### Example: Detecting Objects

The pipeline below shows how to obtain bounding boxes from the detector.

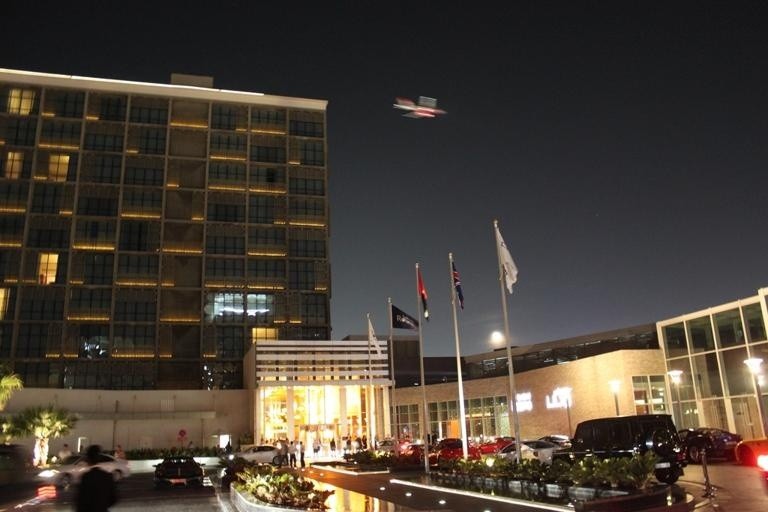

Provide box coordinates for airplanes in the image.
[393,95,448,120]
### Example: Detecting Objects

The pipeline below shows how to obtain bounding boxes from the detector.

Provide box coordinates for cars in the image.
[223,445,288,466]
[402,435,572,466]
[37,452,132,490]
[677,427,742,465]
[153,456,206,489]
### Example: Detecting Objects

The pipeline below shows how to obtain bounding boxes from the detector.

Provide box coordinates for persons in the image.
[299,440,305,468]
[114,443,126,459]
[59,443,72,461]
[65,444,118,512]
[288,440,298,467]
[275,441,289,468]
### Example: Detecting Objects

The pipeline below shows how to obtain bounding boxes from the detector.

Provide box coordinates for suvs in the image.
[574,414,688,485]
[367,440,401,454]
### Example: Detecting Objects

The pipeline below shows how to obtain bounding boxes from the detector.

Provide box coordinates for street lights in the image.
[742,357,768,437]
[558,386,572,435]
[665,368,685,429]
[608,380,620,415]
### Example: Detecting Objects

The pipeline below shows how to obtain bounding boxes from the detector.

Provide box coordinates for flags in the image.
[496,227,519,293]
[391,304,418,331]
[418,269,430,321]
[369,319,380,356]
[452,257,464,310]
